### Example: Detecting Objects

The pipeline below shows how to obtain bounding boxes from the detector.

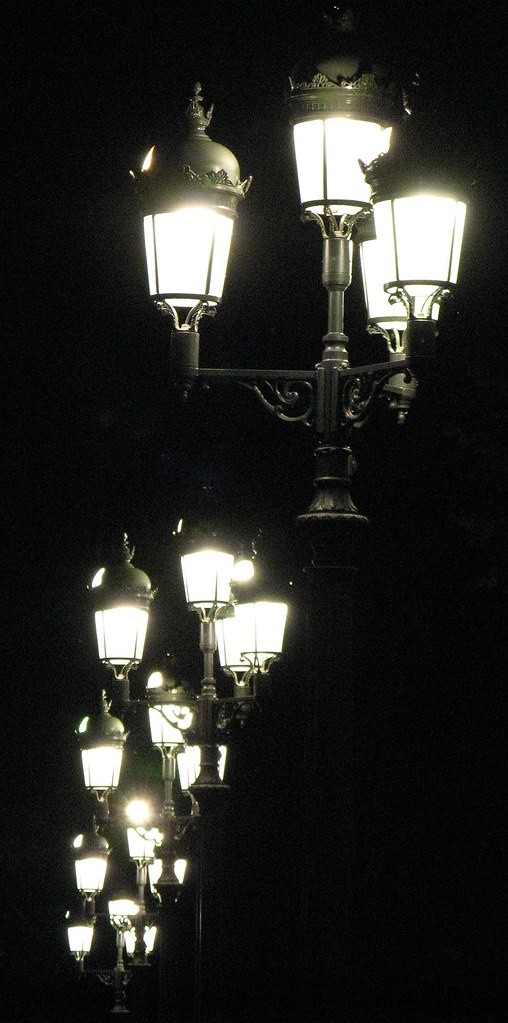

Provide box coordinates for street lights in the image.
[67,9,478,1023]
